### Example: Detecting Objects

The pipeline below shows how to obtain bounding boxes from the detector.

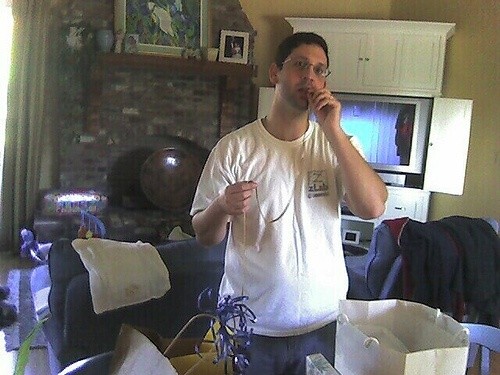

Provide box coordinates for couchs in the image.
[345,217,500,300]
[30,241,228,375]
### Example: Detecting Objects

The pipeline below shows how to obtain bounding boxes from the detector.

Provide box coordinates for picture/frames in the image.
[218,30,250,64]
[113,0,208,59]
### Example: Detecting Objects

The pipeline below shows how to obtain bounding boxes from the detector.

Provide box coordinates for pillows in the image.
[49,239,87,322]
[364,223,402,298]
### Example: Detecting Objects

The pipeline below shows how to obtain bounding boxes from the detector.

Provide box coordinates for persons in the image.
[189,32,388,375]
[227,38,242,60]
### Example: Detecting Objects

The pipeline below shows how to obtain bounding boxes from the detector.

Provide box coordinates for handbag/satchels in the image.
[337,299,469,375]
[109,313,234,375]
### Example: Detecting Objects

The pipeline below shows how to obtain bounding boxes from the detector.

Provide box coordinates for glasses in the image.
[283,58,331,80]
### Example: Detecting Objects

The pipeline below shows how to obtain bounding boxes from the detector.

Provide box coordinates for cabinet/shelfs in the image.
[258,17,474,258]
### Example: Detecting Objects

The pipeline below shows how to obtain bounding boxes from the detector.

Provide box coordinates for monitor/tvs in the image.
[309,92,433,188]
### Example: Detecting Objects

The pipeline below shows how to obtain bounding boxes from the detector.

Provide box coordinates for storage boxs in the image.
[306,353,341,375]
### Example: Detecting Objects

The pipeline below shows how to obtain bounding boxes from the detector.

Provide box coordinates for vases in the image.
[96,21,114,51]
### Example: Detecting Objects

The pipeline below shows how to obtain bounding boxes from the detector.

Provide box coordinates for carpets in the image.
[3,269,48,352]
